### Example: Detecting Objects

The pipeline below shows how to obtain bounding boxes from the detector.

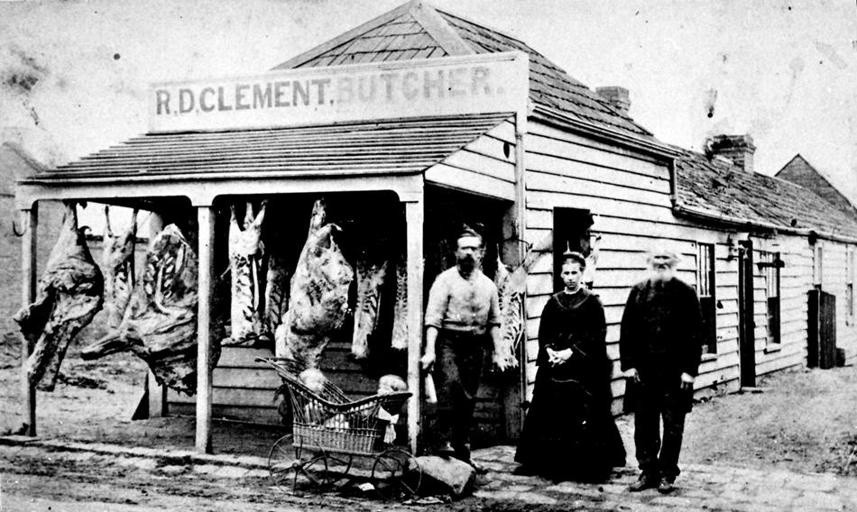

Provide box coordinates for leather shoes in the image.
[631,471,658,492]
[468,459,489,474]
[438,442,455,456]
[659,476,673,492]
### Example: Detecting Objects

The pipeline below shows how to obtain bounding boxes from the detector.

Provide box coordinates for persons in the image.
[619,243,705,494]
[513,252,626,485]
[417,231,514,462]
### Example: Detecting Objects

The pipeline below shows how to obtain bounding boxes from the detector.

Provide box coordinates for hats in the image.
[562,251,586,268]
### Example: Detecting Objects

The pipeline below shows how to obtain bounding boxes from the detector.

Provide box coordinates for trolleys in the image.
[255,356,422,501]
